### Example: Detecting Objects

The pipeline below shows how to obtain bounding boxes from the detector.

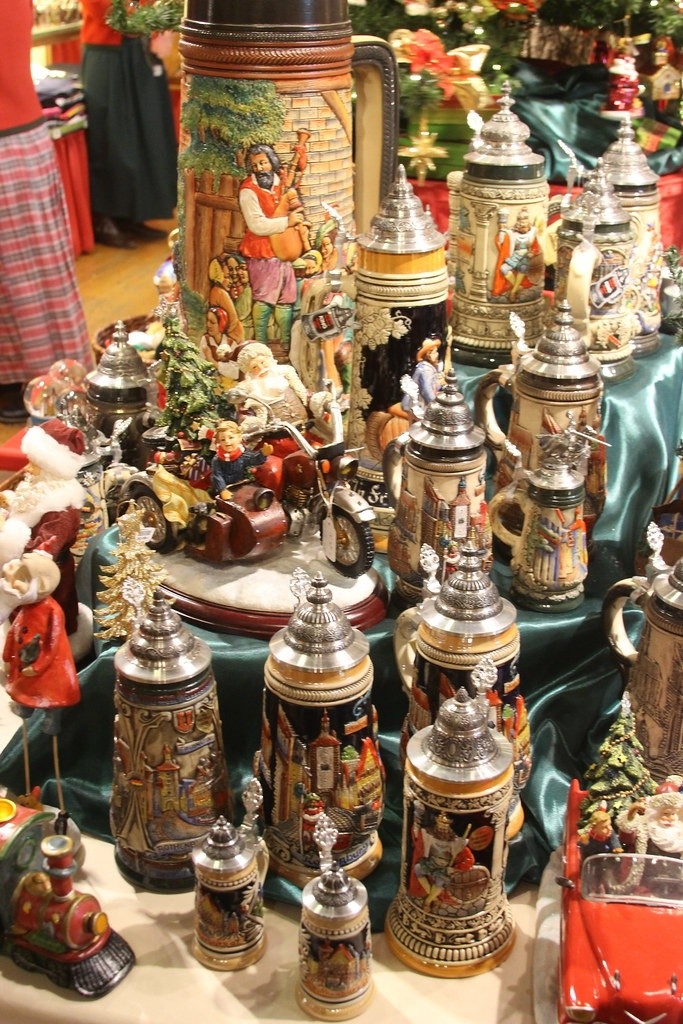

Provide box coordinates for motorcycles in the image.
[117,378,378,580]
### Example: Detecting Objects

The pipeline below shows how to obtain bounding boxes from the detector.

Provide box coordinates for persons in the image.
[1,552,80,736]
[76,1,179,249]
[401,338,445,412]
[606,49,642,112]
[221,338,336,450]
[1,1,98,426]
[617,773,683,901]
[1,416,89,642]
[210,420,274,499]
[575,810,624,895]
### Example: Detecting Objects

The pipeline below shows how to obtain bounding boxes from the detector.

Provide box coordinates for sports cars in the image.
[555,780,683,1024]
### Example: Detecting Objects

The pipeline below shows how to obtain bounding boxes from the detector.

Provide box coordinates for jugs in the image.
[87,0,683,977]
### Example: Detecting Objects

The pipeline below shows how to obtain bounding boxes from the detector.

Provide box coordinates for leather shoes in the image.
[94,215,137,247]
[0,391,30,424]
[113,215,167,240]
